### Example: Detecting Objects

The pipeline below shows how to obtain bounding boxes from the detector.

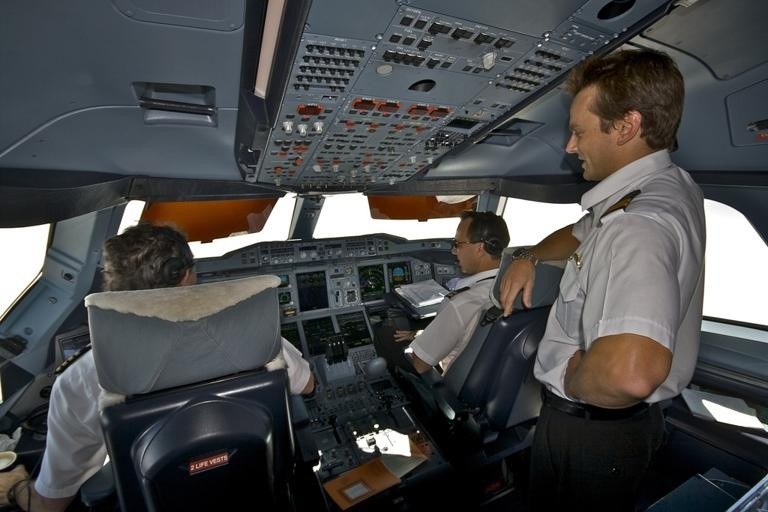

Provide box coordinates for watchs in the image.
[512,248,538,266]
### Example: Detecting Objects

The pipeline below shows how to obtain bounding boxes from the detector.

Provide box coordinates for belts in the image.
[544,389,650,420]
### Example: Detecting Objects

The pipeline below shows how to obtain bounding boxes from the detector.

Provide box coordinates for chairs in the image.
[642,418,768,511]
[77,276,305,512]
[399,247,570,506]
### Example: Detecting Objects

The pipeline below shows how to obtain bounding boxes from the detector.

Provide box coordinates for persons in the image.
[499,48,706,512]
[0,223,314,512]
[393,211,510,374]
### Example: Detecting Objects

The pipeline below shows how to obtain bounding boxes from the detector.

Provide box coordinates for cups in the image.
[0,452,17,472]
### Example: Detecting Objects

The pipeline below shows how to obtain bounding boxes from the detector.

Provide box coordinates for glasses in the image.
[451,239,485,248]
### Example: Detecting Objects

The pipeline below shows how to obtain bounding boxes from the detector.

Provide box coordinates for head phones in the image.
[484,211,503,256]
[162,257,187,284]
